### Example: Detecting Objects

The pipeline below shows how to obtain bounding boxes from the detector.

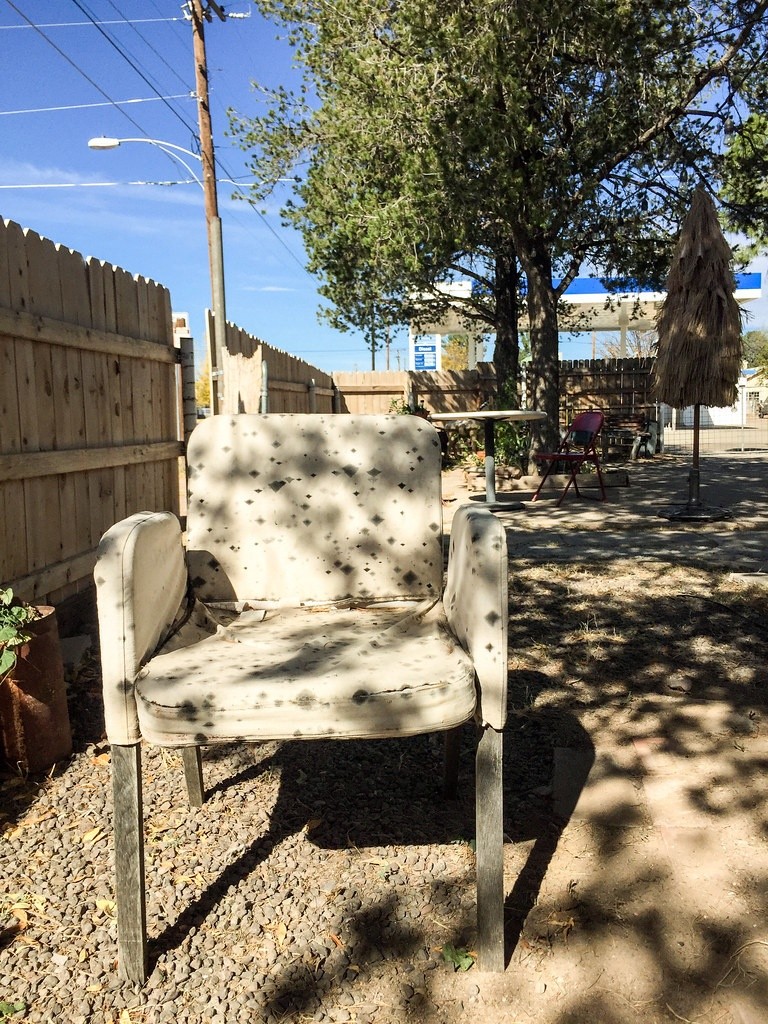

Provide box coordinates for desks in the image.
[431,410,546,511]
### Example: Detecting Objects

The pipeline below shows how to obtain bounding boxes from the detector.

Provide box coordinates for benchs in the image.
[602,412,648,458]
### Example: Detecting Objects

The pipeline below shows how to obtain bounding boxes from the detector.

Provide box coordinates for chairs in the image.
[93,414,509,986]
[533,412,606,506]
